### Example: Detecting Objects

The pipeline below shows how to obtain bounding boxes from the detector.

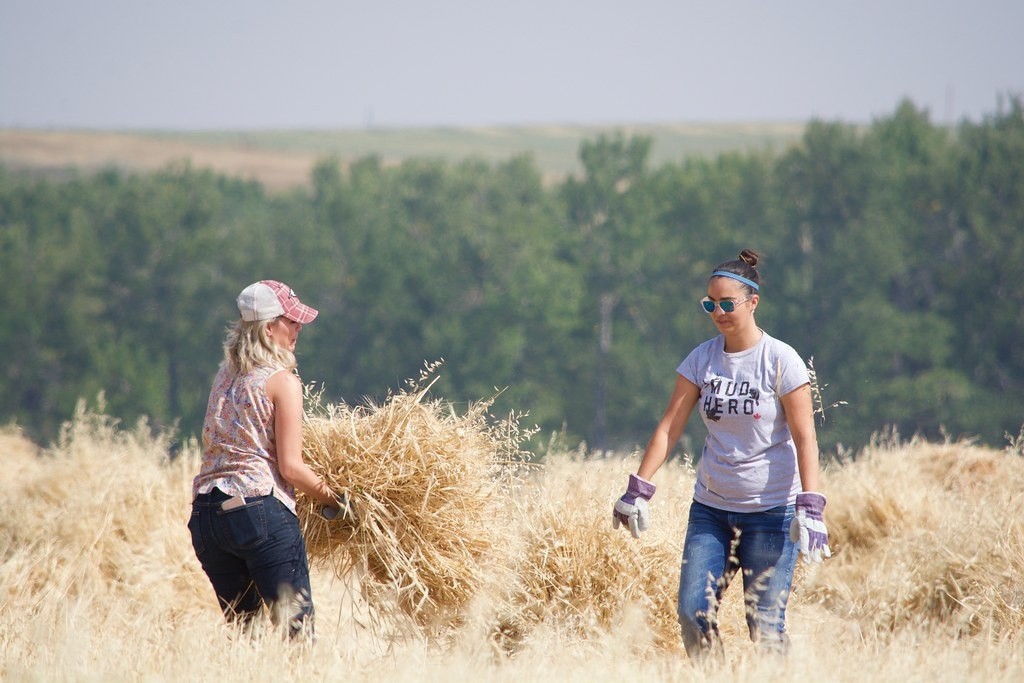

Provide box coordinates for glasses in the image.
[701,295,753,313]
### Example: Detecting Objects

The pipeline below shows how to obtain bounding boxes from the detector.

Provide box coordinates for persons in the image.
[187,281,379,648]
[613,249,832,668]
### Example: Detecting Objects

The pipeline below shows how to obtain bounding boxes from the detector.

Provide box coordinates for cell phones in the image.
[221,495,246,511]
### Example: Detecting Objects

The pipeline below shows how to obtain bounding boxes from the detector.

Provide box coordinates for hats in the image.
[237,280,319,324]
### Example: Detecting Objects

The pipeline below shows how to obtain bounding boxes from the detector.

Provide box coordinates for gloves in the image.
[789,492,831,565]
[613,474,657,539]
[323,494,351,521]
